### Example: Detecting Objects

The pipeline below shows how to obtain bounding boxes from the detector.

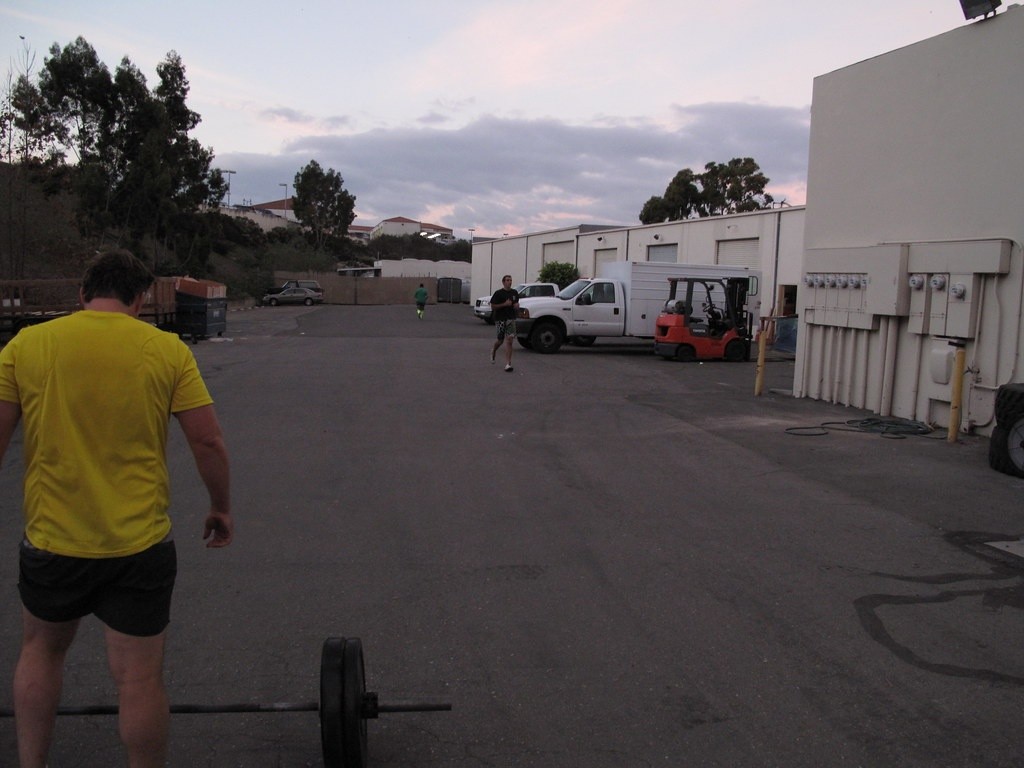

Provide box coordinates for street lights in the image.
[280,181,288,218]
[468,228,475,243]
[219,168,238,207]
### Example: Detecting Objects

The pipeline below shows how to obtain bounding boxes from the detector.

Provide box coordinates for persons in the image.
[488,275,521,371]
[413,282,428,321]
[0,247,237,767]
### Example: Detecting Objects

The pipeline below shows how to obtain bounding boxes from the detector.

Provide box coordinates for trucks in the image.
[517,259,762,354]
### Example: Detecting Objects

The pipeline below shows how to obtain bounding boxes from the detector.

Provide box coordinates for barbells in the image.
[0,632,455,768]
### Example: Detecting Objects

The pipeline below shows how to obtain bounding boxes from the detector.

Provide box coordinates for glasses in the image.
[507,280,512,281]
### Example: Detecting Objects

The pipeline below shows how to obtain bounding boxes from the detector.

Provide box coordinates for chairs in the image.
[689,306,703,324]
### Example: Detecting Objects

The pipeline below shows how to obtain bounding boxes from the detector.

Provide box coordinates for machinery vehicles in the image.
[655,274,799,362]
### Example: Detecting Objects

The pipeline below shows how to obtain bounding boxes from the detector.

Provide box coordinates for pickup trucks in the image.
[475,282,560,325]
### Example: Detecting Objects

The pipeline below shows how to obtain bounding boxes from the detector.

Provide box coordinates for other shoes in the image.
[504,364,513,371]
[491,351,496,364]
[419,312,421,318]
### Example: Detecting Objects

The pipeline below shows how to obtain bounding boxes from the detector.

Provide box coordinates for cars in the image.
[278,280,322,289]
[262,288,324,306]
